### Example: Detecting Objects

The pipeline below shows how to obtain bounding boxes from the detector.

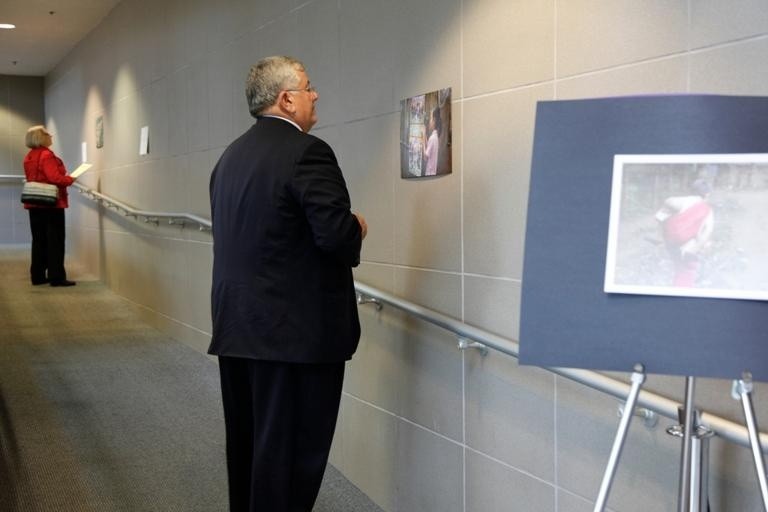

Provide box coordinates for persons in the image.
[655,175,717,289]
[206,54,368,512]
[422,104,443,178]
[21,125,78,287]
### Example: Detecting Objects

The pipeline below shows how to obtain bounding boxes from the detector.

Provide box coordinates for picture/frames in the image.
[603,147,768,304]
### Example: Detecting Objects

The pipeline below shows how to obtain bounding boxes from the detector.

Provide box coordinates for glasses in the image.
[282,82,319,95]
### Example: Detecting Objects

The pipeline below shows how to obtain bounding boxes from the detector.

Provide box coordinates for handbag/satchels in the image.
[19,180,60,205]
